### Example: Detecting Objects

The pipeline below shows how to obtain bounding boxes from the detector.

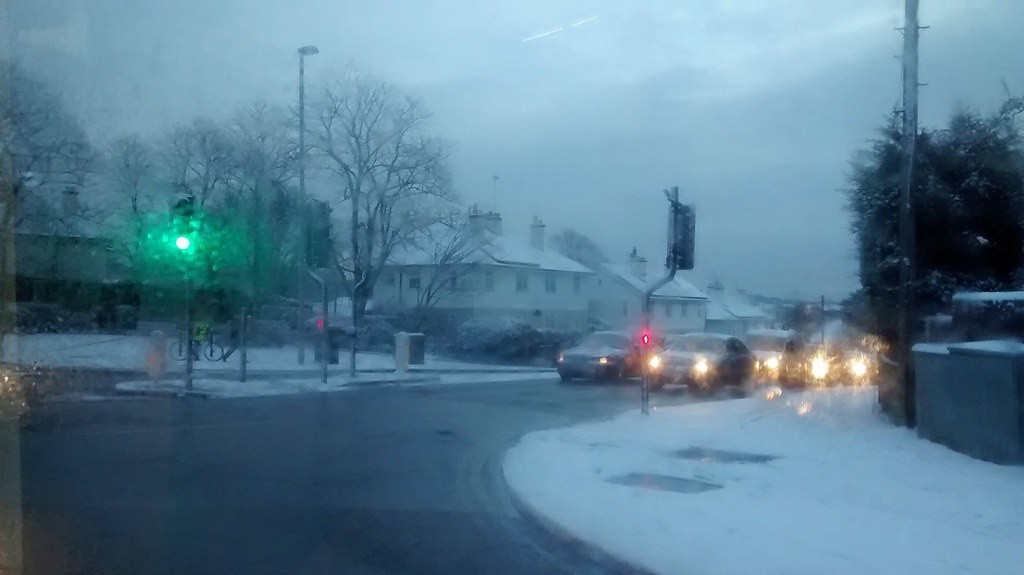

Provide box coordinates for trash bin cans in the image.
[394,333,425,363]
[315,326,339,364]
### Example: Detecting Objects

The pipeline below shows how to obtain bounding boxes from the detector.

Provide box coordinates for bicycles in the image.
[169,328,225,363]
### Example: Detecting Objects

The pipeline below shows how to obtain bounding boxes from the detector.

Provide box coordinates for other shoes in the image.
[220,354,227,363]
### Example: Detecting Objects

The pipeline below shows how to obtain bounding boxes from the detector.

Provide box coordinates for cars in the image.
[649,333,757,395]
[557,331,645,382]
[742,329,878,389]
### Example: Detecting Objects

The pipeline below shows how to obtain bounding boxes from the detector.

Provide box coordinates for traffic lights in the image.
[174,194,195,253]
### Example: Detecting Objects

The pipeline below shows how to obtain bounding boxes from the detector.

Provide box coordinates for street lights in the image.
[298,45,320,365]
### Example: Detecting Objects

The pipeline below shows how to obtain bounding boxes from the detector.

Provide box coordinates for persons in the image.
[221,308,251,363]
[193,317,207,361]
